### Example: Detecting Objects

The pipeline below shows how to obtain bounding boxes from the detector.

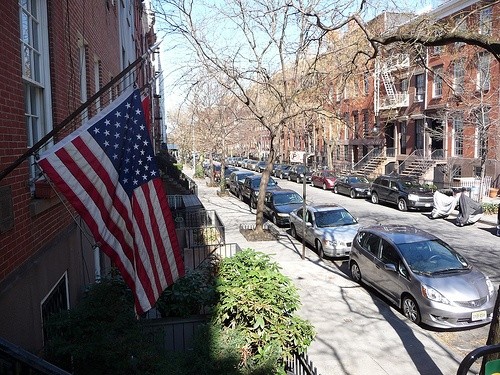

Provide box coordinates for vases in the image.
[489,188,499,198]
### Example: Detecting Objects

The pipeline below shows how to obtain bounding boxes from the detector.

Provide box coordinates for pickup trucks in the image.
[238,175,282,209]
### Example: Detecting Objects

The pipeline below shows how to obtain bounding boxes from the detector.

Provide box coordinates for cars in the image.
[255,161,267,173]
[240,159,252,169]
[311,170,340,190]
[229,171,255,197]
[247,160,260,171]
[263,189,309,227]
[265,164,278,176]
[289,203,363,259]
[287,165,315,183]
[187,155,239,188]
[333,175,372,199]
[274,164,291,180]
[204,152,242,167]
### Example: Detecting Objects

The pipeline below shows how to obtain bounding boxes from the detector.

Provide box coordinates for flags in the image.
[38,86,187,316]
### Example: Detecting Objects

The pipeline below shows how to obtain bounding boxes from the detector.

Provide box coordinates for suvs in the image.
[370,175,434,211]
[348,224,498,329]
